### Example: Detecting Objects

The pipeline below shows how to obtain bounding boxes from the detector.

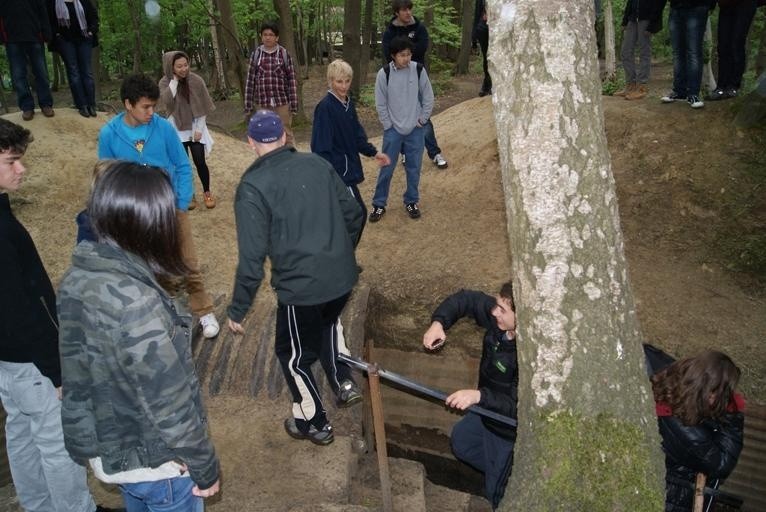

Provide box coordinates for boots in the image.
[612,82,649,100]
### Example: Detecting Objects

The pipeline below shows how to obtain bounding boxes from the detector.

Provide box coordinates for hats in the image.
[250,110,285,143]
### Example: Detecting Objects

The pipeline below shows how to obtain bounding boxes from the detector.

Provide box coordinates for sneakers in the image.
[660,86,736,108]
[203,192,216,208]
[369,207,385,221]
[23,110,34,120]
[41,106,54,117]
[336,380,364,409]
[406,202,421,218]
[285,416,334,446]
[433,154,447,169]
[200,312,220,338]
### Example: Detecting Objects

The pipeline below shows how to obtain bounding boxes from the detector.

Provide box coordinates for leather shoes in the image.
[80,103,96,117]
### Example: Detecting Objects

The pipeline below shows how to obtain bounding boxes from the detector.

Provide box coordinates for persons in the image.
[309,61,390,273]
[1,0,100,121]
[369,39,434,223]
[245,23,299,152]
[704,0,766,101]
[423,280,519,507]
[56,157,221,510]
[1,118,97,510]
[159,52,216,209]
[471,0,492,97]
[660,0,717,108]
[76,160,115,245]
[381,2,448,169]
[99,73,221,339]
[644,342,744,512]
[226,110,365,445]
[613,0,667,100]
